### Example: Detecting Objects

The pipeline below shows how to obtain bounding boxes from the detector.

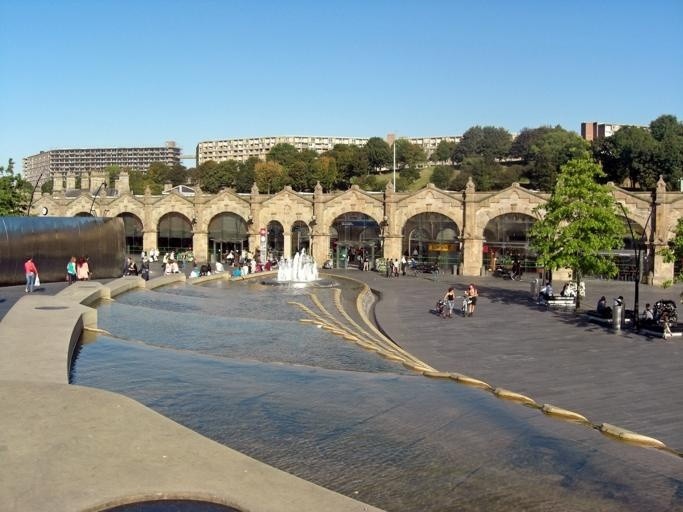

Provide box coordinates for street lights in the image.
[613,202,661,332]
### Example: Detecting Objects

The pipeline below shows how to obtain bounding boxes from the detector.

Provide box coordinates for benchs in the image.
[635,316,683,340]
[583,307,638,330]
[536,291,577,309]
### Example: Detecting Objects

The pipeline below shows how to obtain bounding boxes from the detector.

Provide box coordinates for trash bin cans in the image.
[530,278,544,299]
[613,298,625,330]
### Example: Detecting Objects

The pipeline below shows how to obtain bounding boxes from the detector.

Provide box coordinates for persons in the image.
[598,295,612,314]
[560,282,585,302]
[346,245,439,279]
[444,287,457,318]
[537,283,553,306]
[125,245,280,281]
[642,303,653,320]
[66,254,93,285]
[24,256,39,292]
[511,253,524,282]
[464,283,477,317]
[614,295,624,306]
[659,310,672,340]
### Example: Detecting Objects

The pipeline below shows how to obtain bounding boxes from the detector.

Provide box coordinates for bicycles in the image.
[459,295,471,317]
[435,299,450,319]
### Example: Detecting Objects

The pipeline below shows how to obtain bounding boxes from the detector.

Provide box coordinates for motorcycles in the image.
[491,260,503,277]
[501,268,521,281]
